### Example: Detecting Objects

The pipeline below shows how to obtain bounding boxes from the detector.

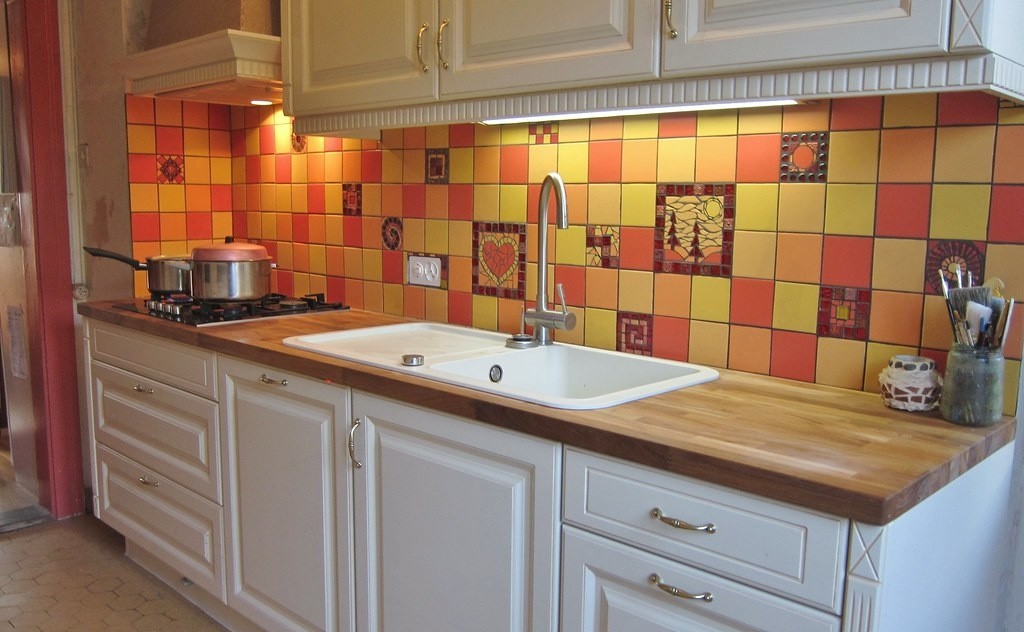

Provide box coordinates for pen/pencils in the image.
[938,265,1015,349]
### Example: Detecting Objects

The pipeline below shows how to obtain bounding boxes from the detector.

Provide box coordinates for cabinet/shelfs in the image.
[80,316,228,628]
[563,443,1014,632]
[218,352,355,632]
[349,385,563,632]
[280,0,663,136]
[659,1,1023,114]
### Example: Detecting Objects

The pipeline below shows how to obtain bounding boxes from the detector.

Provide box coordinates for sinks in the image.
[281,323,721,411]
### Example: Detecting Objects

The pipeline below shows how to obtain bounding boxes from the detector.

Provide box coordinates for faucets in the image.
[522,171,577,346]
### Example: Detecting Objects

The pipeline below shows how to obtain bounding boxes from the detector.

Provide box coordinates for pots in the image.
[189,236,277,301]
[83,246,192,292]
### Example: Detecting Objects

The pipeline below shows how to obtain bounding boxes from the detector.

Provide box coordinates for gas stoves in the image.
[113,293,350,328]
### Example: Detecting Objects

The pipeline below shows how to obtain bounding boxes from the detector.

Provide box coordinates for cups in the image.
[940,343,1004,427]
